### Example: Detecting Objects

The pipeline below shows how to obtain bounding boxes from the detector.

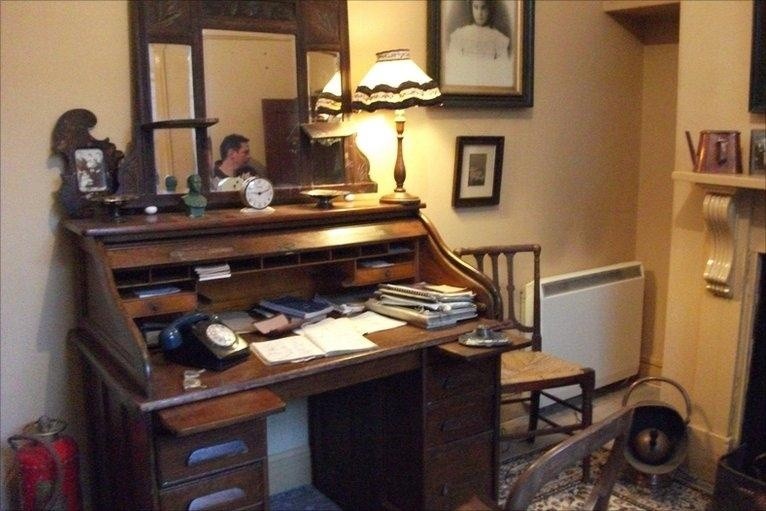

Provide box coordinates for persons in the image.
[213,134,257,182]
[78,149,105,191]
[163,173,178,192]
[447,1,513,59]
[177,173,208,220]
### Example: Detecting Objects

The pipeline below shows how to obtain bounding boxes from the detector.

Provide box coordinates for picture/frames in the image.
[451,134,505,208]
[427,1,535,108]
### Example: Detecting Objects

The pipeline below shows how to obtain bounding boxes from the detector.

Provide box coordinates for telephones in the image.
[158,312,250,371]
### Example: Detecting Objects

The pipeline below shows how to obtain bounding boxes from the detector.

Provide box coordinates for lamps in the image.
[351,48,444,204]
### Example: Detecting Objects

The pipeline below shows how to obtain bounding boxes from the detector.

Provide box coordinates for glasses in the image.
[182,368,207,389]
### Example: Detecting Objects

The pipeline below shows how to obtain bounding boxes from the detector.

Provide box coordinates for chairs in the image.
[441,407,637,511]
[451,242,596,485]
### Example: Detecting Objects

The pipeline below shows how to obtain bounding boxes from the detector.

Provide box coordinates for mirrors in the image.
[104,1,378,217]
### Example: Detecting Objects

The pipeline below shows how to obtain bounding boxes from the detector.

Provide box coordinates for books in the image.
[137,312,180,347]
[357,259,396,269]
[191,260,233,282]
[130,285,182,300]
[212,282,482,367]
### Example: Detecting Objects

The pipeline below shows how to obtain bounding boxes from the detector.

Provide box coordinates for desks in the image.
[66,192,538,511]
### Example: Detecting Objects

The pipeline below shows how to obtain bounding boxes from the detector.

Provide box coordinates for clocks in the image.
[239,175,276,214]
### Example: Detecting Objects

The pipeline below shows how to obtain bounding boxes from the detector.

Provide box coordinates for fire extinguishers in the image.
[8,415,84,511]
